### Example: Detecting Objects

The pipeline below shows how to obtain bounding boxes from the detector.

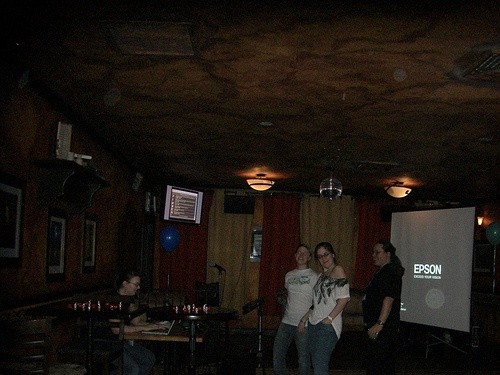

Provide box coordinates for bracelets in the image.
[377,320,384,326]
[327,315,334,321]
[300,318,306,323]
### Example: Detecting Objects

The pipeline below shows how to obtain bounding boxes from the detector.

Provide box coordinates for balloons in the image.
[159,226,180,252]
[485,221,500,246]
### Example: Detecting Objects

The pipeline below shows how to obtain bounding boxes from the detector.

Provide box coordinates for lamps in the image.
[246,174,275,191]
[385,182,412,198]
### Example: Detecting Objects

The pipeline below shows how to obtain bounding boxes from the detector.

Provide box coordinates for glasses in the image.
[318,251,332,260]
[126,281,141,287]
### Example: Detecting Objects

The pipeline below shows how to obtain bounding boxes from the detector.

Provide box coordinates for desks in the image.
[26,303,132,375]
[123,322,210,375]
[151,305,237,375]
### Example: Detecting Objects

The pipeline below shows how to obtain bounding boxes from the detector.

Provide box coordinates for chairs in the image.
[56,308,126,375]
[0,318,87,375]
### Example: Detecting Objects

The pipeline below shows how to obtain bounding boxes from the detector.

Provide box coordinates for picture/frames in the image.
[0,172,27,269]
[80,212,97,274]
[46,207,67,284]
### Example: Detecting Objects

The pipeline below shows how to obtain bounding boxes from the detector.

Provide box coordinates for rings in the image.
[376,333,378,334]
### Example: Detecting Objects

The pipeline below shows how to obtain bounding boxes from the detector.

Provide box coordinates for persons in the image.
[297,242,350,375]
[273,244,346,375]
[362,240,405,375]
[101,269,171,375]
